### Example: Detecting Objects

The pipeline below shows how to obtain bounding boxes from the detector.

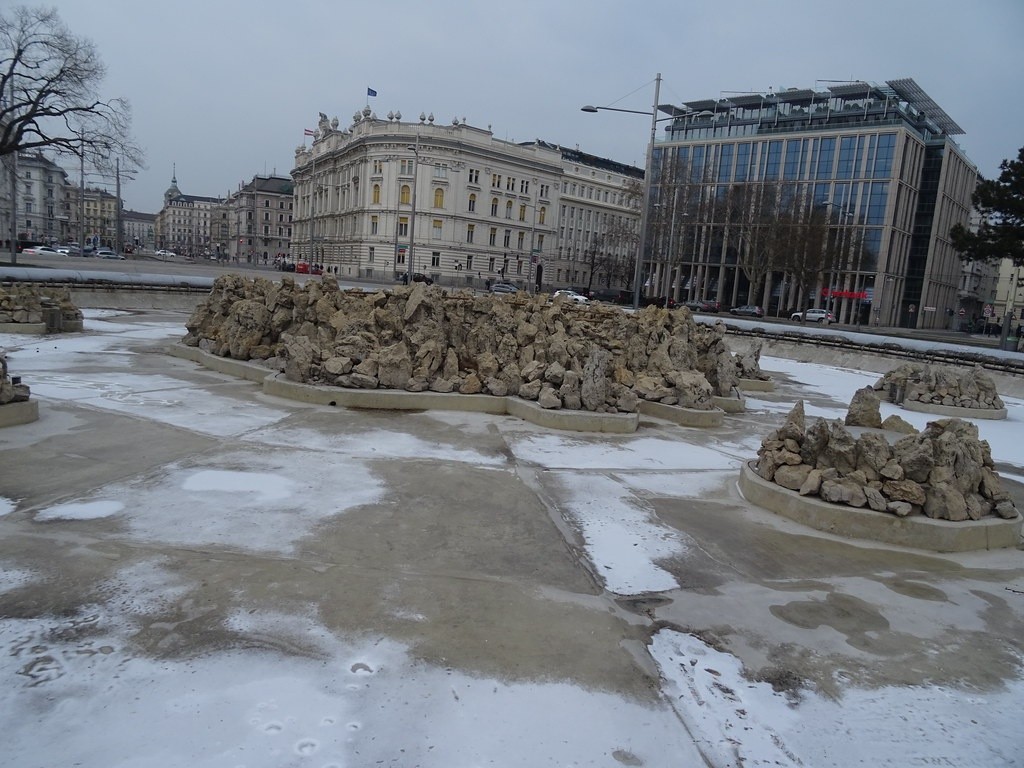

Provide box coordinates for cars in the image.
[94,251,126,260]
[156,250,176,258]
[730,305,764,319]
[553,290,588,305]
[791,309,836,324]
[11,239,69,257]
[403,272,434,286]
[674,299,719,313]
[489,284,519,294]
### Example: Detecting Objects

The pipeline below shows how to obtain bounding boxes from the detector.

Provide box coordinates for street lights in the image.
[408,133,460,286]
[240,239,243,244]
[35,128,138,257]
[581,73,715,309]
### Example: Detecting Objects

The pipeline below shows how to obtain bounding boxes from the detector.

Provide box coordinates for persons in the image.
[988,323,998,338]
[403,273,408,285]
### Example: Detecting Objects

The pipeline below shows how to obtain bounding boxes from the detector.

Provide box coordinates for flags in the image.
[368,88,376,97]
[305,129,313,136]
